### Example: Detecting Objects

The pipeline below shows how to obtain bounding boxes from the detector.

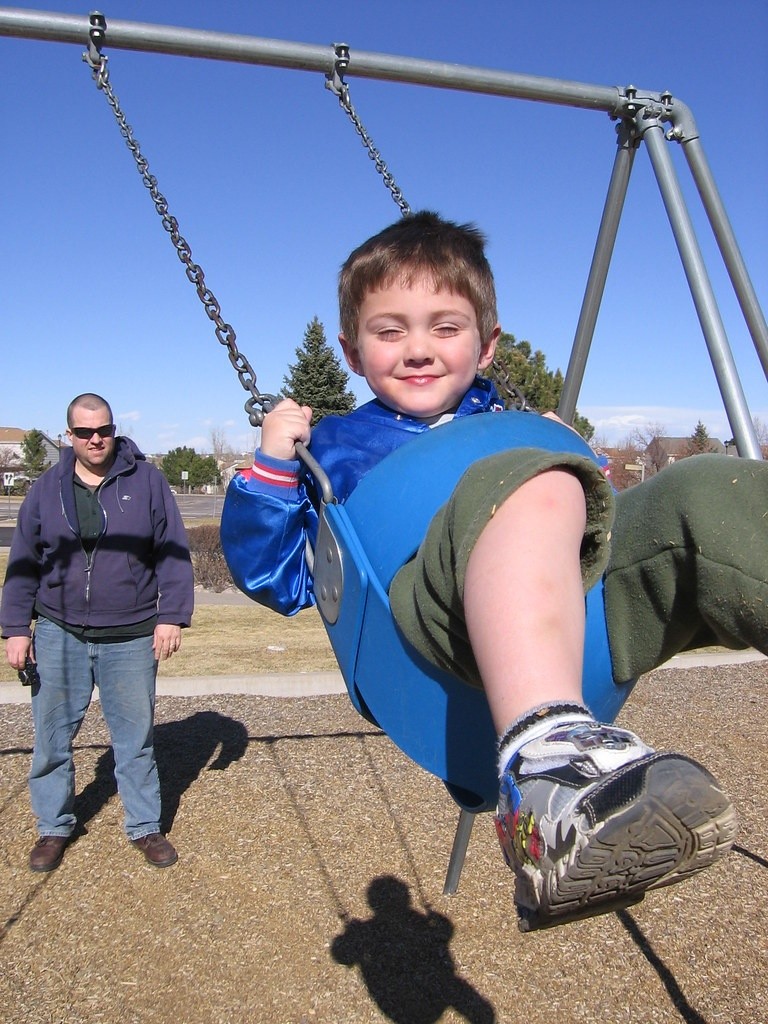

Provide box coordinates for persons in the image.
[0,393,194,872]
[219,210,768,933]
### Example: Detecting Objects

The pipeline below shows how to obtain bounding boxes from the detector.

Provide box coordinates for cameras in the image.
[18,656,39,687]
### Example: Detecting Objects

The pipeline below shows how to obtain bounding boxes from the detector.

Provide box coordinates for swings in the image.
[94,53,640,793]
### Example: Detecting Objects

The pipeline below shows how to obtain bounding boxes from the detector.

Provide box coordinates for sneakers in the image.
[30,835,68,871]
[131,832,177,867]
[495,717,738,933]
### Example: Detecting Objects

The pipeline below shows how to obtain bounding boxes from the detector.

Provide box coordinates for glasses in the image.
[70,424,116,440]
